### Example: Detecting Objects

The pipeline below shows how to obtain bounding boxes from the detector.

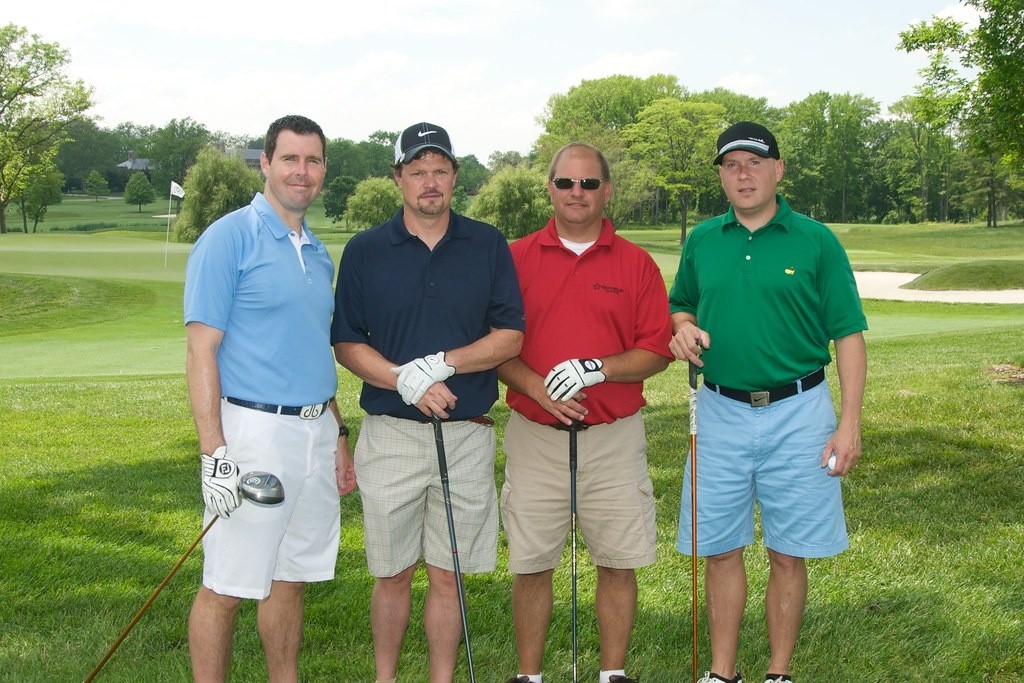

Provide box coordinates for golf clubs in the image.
[566,418,583,682]
[78,470,287,683]
[429,409,476,683]
[686,342,702,682]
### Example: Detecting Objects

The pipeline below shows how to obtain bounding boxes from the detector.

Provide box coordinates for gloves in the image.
[544,358,607,402]
[200,446,242,519]
[391,350,456,406]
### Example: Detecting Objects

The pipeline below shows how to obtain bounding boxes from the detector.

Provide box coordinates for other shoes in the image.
[504,675,543,683]
[599,674,641,683]
[696,670,742,683]
[764,677,792,683]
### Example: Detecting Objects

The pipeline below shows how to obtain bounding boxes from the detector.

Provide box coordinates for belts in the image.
[384,414,494,427]
[548,417,596,431]
[221,396,336,419]
[703,365,825,407]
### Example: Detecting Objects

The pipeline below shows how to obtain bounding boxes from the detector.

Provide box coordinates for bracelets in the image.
[338,425,349,438]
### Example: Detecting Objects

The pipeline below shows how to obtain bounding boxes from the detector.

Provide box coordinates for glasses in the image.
[549,177,609,190]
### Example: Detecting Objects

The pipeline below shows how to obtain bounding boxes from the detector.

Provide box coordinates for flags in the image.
[170,182,186,200]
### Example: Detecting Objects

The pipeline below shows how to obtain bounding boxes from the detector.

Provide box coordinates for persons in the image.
[331,124,525,683]
[183,116,357,683]
[496,141,677,683]
[669,122,869,683]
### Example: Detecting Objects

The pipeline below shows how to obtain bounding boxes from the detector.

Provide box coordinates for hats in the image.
[390,123,458,168]
[713,121,780,167]
